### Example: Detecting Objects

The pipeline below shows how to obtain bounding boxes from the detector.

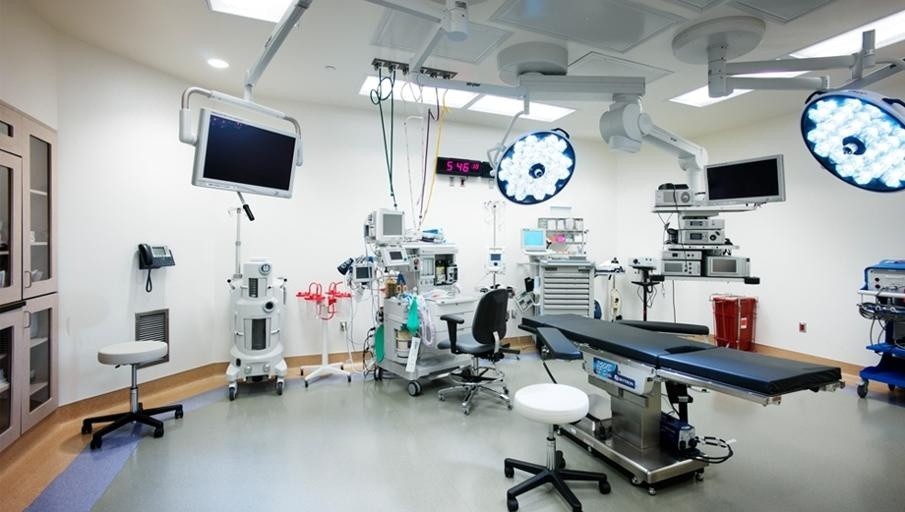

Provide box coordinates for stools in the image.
[81,340,184,450]
[505,384,611,512]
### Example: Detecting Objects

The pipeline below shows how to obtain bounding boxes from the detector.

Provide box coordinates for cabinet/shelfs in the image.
[1,99,58,454]
[537,217,587,260]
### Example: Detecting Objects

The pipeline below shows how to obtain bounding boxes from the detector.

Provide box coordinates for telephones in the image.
[139,244,176,272]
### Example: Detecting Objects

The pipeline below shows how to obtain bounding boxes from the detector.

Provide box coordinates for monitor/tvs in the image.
[192,107,300,200]
[521,228,547,251]
[375,208,406,243]
[703,154,787,205]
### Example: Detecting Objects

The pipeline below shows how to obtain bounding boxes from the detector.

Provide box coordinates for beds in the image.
[518,314,847,496]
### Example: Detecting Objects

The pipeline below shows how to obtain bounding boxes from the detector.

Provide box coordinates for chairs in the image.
[437,288,513,416]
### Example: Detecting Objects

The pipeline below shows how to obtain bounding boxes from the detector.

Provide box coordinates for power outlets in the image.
[340,322,347,332]
